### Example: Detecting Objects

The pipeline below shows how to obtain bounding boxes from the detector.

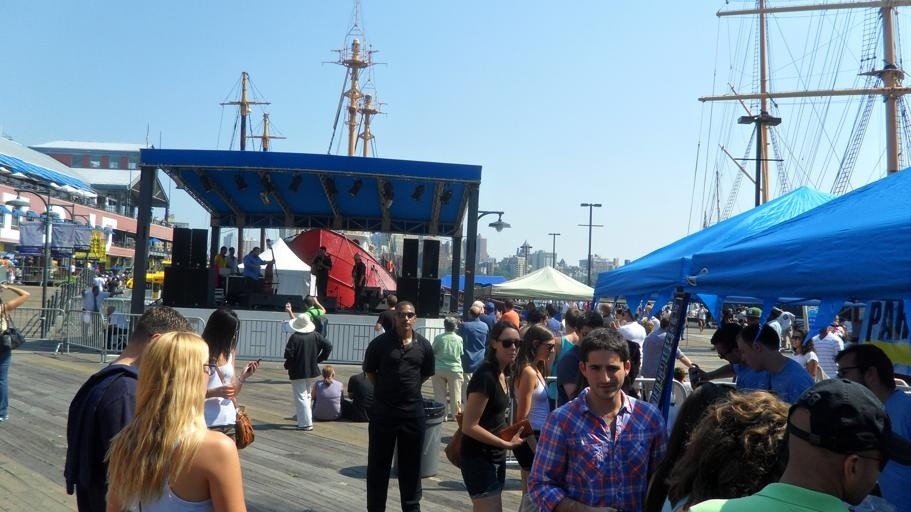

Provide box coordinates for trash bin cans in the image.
[420,401,446,478]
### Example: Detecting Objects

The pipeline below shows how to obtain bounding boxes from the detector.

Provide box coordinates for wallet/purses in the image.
[500,420,532,441]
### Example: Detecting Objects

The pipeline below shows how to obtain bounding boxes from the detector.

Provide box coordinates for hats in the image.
[788,378,911,464]
[743,307,761,318]
[289,314,316,333]
[473,300,496,309]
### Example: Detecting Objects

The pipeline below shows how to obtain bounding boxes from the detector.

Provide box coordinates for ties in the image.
[94,296,97,311]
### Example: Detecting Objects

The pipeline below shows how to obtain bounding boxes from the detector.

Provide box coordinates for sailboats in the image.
[286,0,403,309]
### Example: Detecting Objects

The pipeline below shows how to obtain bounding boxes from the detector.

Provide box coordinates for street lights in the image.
[520,246,535,276]
[47,202,76,282]
[6,186,52,339]
[547,232,562,269]
[577,201,605,287]
[479,210,513,233]
[737,111,785,207]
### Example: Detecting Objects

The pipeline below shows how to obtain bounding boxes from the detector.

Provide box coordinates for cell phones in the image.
[247,358,262,372]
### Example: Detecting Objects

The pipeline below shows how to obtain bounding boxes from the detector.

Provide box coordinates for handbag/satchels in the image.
[237,409,254,448]
[444,413,463,468]
[0,304,25,350]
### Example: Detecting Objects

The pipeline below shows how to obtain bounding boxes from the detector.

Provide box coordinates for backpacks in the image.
[308,309,328,336]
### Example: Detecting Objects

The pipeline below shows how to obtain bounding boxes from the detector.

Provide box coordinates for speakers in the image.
[422,240,440,278]
[264,294,306,313]
[419,277,441,317]
[318,296,338,314]
[164,267,216,308]
[402,239,419,277]
[172,228,190,266]
[191,229,207,267]
[397,277,420,318]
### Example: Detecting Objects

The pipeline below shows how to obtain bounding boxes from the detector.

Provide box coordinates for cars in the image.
[127,258,173,289]
[841,302,869,341]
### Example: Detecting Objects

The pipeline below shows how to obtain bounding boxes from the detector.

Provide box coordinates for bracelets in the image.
[239,379,246,384]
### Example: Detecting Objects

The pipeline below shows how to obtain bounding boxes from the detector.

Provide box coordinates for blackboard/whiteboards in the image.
[651,291,691,412]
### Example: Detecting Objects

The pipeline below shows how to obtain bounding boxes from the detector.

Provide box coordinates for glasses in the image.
[399,313,415,318]
[498,339,522,348]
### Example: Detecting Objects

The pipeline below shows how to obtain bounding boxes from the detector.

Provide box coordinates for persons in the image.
[0,283,32,422]
[98,330,250,511]
[310,364,345,422]
[515,326,552,510]
[688,377,893,510]
[210,234,371,313]
[65,302,194,510]
[362,300,423,511]
[430,315,462,422]
[526,327,669,511]
[79,264,163,350]
[374,294,400,335]
[646,380,793,510]
[457,321,514,511]
[462,300,849,412]
[7,265,23,284]
[346,363,376,423]
[833,343,910,510]
[199,308,263,447]
[285,293,327,339]
[283,312,333,431]
